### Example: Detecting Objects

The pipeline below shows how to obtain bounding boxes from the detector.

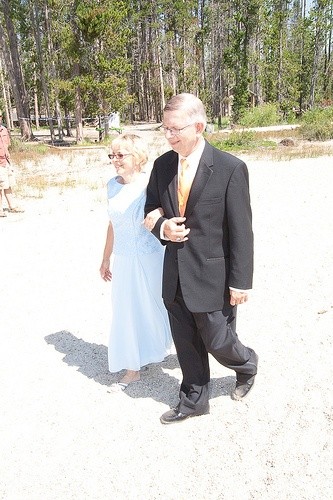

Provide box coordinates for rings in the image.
[177,238,181,241]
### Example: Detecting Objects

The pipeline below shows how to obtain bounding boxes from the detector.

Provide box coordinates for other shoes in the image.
[8,206,25,212]
[0,210,6,218]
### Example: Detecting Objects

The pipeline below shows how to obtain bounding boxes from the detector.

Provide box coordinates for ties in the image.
[178,159,186,216]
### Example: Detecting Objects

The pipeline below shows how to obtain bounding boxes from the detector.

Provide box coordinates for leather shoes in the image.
[160,406,209,424]
[230,353,258,401]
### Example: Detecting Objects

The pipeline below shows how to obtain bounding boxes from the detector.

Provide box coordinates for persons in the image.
[144,93,258,425]
[0,115,24,217]
[100,133,164,393]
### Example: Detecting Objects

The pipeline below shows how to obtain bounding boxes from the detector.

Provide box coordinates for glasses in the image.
[159,123,191,135]
[108,153,133,159]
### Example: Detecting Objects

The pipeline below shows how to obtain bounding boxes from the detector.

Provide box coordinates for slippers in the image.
[109,379,133,392]
